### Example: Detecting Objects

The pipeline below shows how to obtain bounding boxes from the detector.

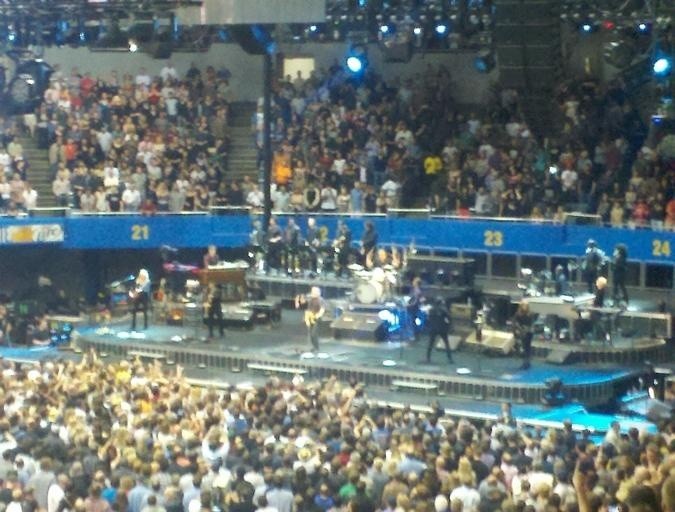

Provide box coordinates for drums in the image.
[356,281,383,303]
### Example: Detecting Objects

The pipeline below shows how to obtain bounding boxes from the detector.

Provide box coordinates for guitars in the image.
[128,281,147,302]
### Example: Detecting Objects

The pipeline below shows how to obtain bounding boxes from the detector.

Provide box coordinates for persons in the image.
[0,0,675,511]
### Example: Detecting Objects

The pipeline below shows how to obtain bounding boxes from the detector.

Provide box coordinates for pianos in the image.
[198,259,248,301]
[529,293,596,342]
